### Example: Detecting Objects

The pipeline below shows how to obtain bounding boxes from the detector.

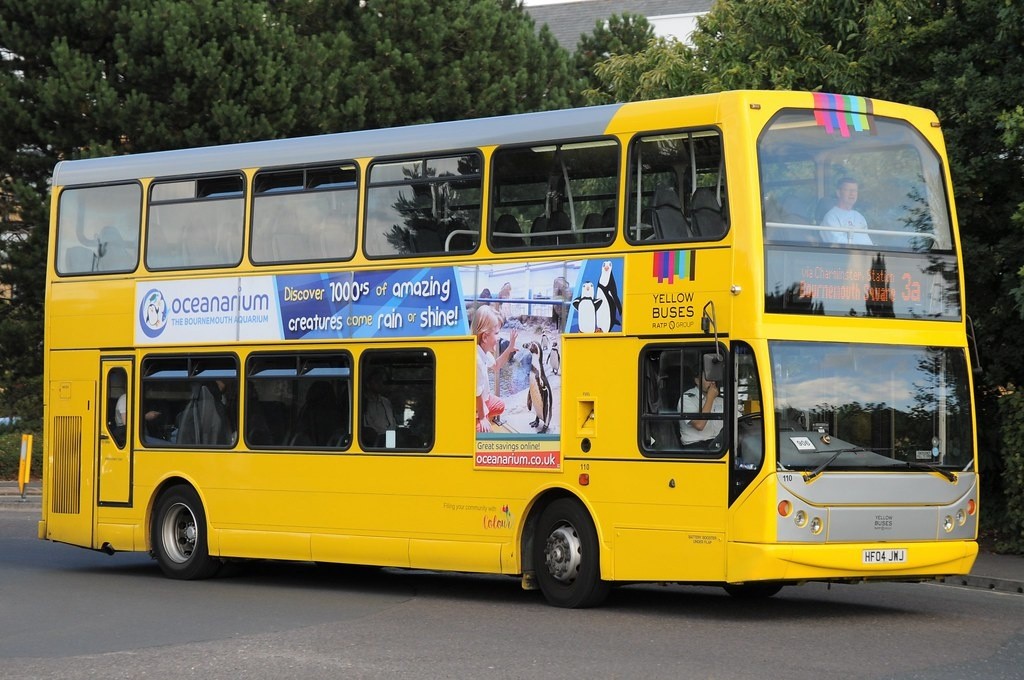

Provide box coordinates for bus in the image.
[37,88,987,609]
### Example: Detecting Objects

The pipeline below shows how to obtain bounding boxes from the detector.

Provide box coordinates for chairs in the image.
[65,187,836,273]
[110,395,434,451]
[642,375,683,452]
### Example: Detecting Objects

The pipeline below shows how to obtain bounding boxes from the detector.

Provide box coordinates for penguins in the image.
[522,331,560,434]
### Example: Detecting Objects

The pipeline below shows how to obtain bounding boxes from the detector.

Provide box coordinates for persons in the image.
[469,303,520,434]
[114,361,435,450]
[676,365,741,450]
[818,175,875,246]
